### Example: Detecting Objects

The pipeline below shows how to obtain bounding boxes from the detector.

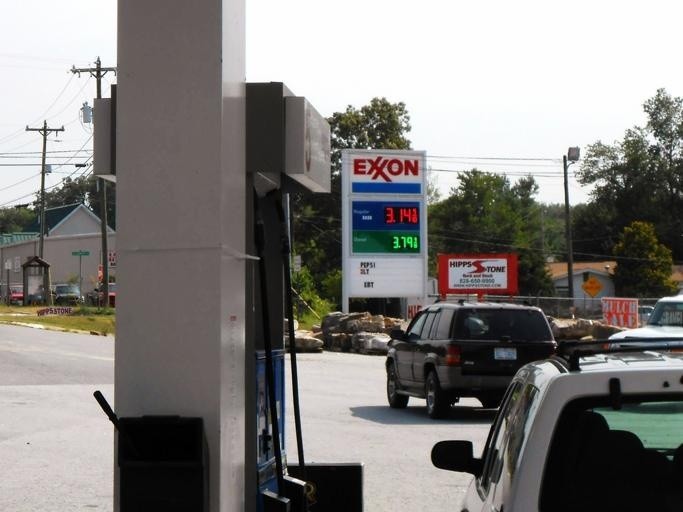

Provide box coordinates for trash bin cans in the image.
[116,414,210,512]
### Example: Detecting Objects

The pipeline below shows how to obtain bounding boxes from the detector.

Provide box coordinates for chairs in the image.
[587,412,644,449]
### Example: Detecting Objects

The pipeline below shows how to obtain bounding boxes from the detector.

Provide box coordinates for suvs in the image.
[426,334,682,510]
[383,297,557,418]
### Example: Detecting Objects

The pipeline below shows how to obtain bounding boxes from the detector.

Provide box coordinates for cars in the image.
[0,282,117,309]
[603,296,683,352]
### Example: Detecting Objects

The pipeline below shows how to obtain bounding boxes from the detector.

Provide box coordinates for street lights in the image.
[562,146,582,317]
